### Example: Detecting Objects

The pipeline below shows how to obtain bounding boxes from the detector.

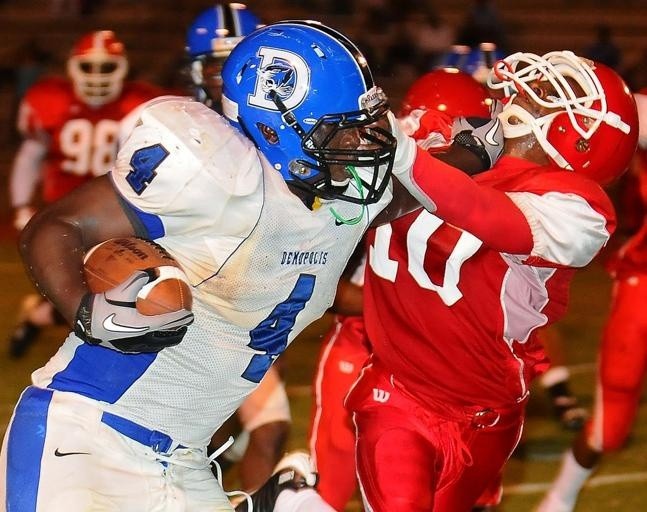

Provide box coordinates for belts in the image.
[100,410,189,469]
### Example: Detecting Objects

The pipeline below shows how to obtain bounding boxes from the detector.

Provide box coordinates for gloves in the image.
[450,98,505,170]
[71,267,195,356]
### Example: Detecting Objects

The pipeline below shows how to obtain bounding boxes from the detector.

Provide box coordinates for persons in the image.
[1,0,646,512]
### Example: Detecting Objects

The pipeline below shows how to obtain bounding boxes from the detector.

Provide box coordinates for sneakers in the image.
[546,380,589,431]
[10,292,45,360]
[234,450,319,512]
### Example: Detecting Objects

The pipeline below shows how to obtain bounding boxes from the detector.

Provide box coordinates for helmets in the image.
[219,19,376,183]
[541,56,639,189]
[64,29,131,60]
[399,65,495,155]
[184,2,269,63]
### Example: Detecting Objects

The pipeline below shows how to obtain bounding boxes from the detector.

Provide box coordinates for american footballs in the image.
[82,235,192,316]
